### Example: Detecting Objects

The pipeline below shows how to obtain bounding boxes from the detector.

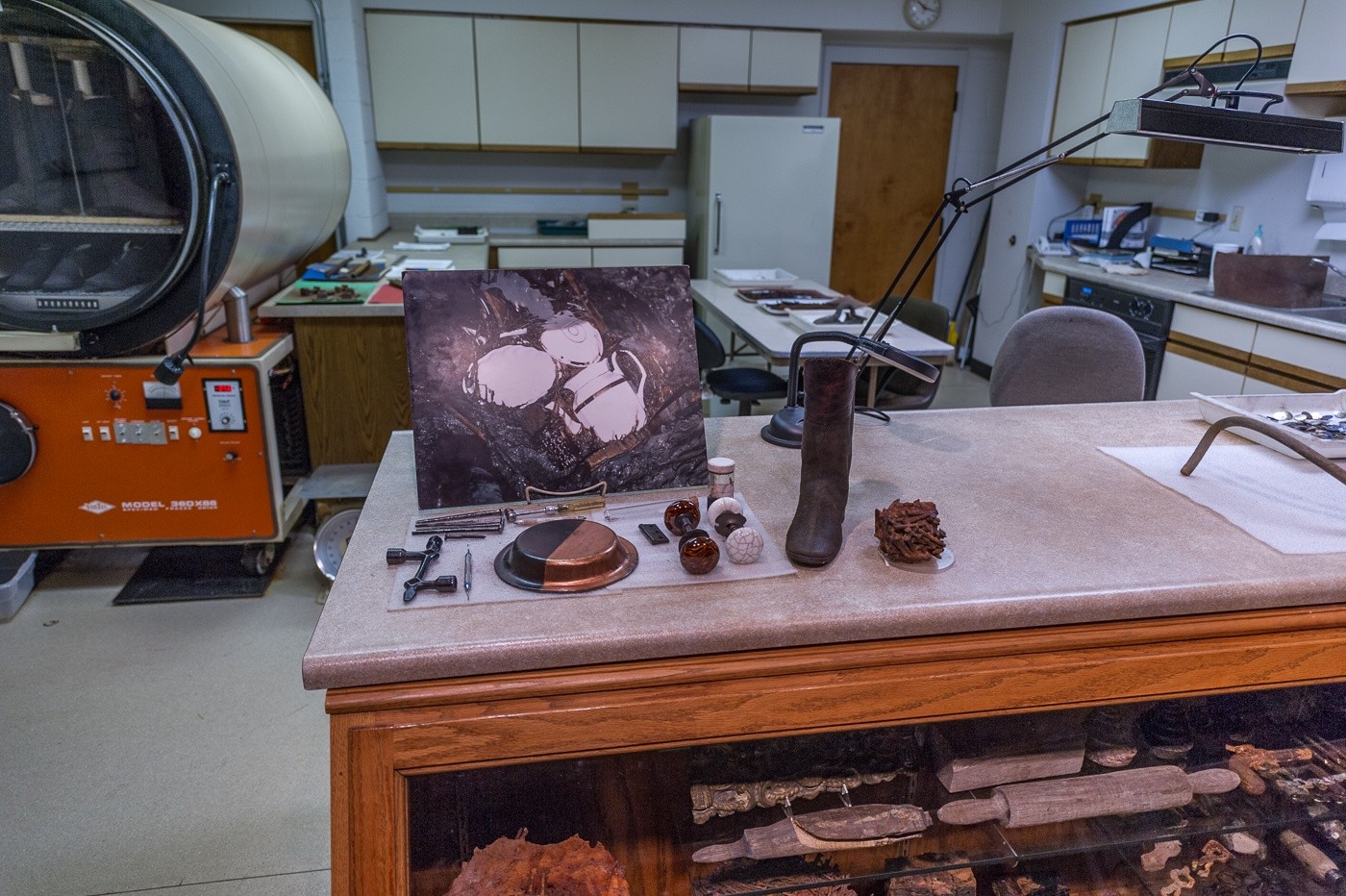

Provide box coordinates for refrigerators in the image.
[688,108,840,291]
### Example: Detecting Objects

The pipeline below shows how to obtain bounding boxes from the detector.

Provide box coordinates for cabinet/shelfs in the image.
[361,8,579,152]
[1153,302,1346,401]
[1284,0,1346,98]
[1167,0,1309,87]
[578,20,680,156]
[300,394,1345,895]
[494,245,687,269]
[679,22,824,95]
[1046,6,1207,170]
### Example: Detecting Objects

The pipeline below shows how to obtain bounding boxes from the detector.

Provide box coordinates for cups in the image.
[1207,242,1240,287]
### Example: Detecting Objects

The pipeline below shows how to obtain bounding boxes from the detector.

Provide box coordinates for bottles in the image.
[1244,225,1264,258]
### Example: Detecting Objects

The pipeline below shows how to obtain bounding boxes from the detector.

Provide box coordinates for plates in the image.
[389,257,452,286]
[782,308,902,335]
[412,226,489,244]
[712,268,798,289]
[738,286,831,303]
[756,297,864,314]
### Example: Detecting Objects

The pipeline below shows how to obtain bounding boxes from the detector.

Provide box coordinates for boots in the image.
[784,355,857,566]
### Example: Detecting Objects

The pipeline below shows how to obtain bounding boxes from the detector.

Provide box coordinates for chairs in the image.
[692,318,789,418]
[848,296,951,409]
[988,306,1145,408]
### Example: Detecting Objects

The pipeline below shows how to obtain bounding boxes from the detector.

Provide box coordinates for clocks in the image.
[903,0,943,30]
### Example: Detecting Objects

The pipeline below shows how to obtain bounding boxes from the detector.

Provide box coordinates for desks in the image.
[689,275,957,422]
